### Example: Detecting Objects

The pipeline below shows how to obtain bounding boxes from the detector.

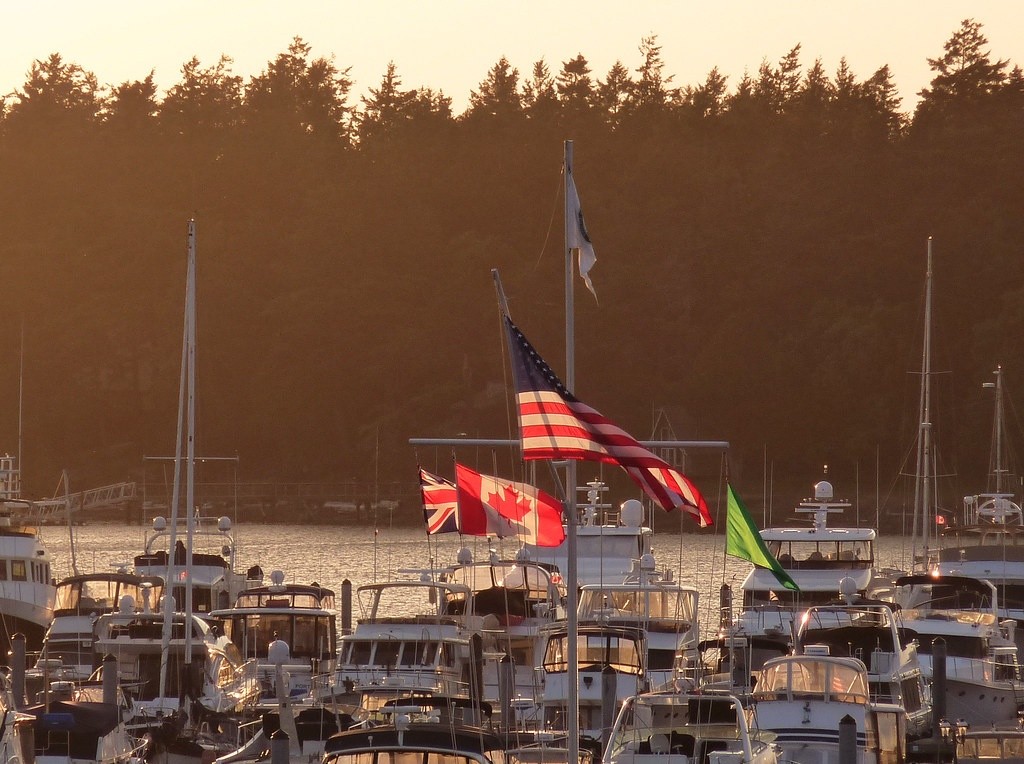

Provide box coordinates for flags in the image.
[722,479,803,594]
[566,171,602,309]
[502,311,714,529]
[415,462,566,548]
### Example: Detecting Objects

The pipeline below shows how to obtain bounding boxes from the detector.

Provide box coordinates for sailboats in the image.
[1,138,1024,764]
